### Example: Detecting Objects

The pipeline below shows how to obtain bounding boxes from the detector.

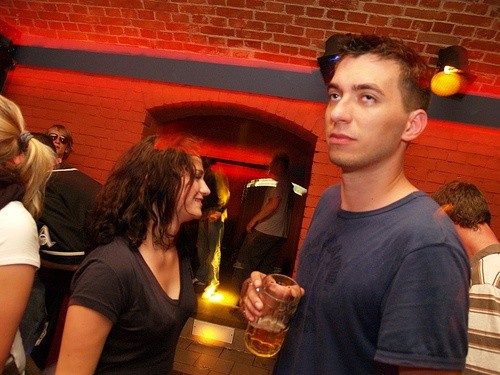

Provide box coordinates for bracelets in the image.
[215,207,224,212]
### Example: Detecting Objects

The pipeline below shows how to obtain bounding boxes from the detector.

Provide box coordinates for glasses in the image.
[49,133,70,144]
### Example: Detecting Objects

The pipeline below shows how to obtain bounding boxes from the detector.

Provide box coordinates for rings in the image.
[248,282,253,286]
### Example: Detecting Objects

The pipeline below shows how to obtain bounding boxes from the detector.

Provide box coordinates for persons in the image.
[239,33,471,375]
[228,155,292,307]
[0,95,58,375]
[431,180,500,375]
[47,124,74,164]
[191,155,230,299]
[15,132,104,373]
[55,135,211,375]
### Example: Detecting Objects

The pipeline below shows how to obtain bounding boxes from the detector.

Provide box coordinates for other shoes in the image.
[233,261,245,269]
[192,277,206,286]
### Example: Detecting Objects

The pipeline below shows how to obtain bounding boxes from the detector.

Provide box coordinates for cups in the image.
[244,273,301,358]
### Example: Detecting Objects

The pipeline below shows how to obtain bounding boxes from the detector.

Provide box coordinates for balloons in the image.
[430,72,460,96]
